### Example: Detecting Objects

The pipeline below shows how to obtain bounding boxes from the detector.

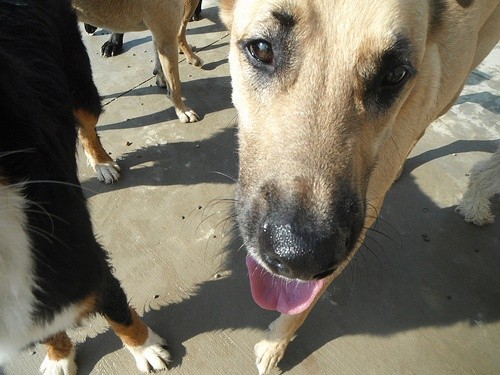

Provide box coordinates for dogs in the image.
[69,0,208,123]
[219,0,499,375]
[0,0,175,374]
